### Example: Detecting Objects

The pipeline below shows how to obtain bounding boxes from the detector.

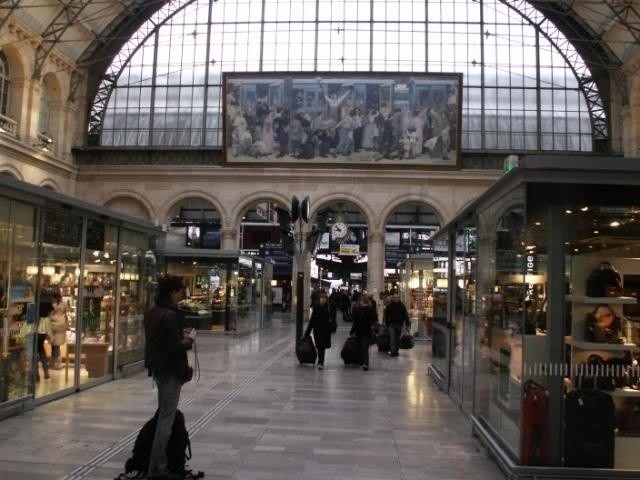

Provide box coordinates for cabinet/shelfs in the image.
[564,255,640,410]
[488,327,552,467]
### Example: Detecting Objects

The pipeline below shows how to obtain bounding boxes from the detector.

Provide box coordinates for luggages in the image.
[562,369,614,467]
[376,326,390,352]
[296,336,316,365]
[341,337,360,365]
[519,378,551,468]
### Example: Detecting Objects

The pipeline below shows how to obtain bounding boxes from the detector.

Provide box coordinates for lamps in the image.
[291,194,310,223]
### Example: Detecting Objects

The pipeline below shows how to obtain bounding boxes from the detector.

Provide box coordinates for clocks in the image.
[332,222,347,238]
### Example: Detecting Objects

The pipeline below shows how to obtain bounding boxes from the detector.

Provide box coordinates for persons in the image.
[143,276,197,480]
[28,293,70,384]
[212,287,220,302]
[280,284,410,371]
[226,84,457,163]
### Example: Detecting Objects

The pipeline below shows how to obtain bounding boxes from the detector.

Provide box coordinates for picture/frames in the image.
[222,72,463,171]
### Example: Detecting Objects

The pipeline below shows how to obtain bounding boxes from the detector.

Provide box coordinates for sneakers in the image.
[361,364,369,370]
[317,364,323,369]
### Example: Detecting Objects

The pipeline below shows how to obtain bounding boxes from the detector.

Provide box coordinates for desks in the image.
[65,343,109,382]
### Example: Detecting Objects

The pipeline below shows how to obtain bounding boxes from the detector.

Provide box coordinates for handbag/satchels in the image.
[586,260,625,297]
[399,336,415,349]
[126,409,189,474]
[585,304,629,343]
[576,353,639,391]
